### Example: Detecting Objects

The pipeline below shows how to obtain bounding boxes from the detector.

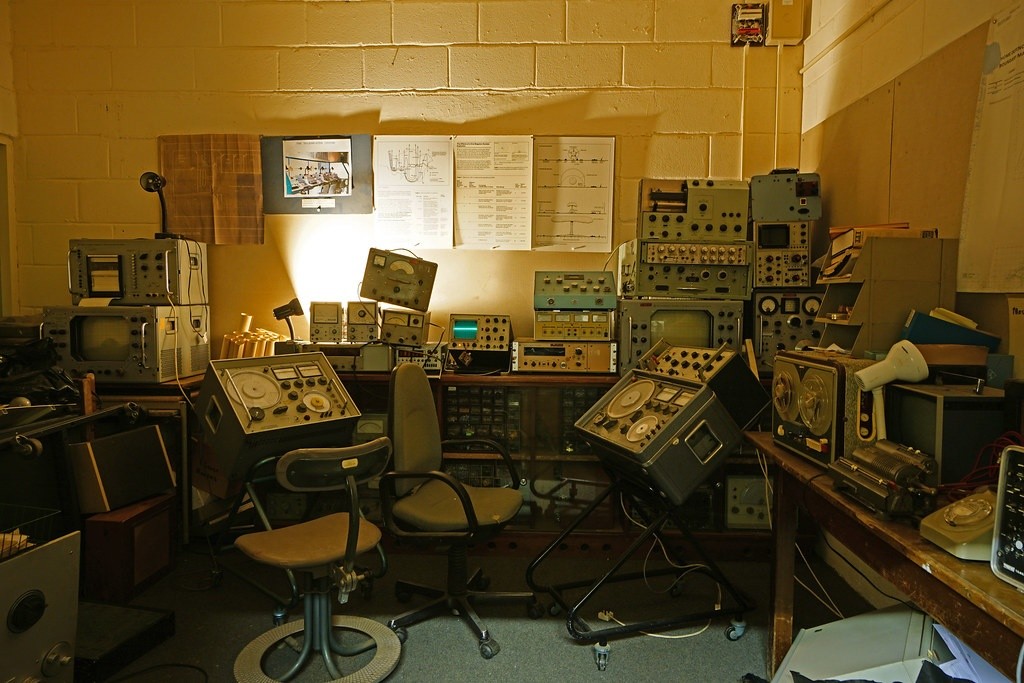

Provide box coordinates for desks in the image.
[743,429,1021,683]
[94,373,209,547]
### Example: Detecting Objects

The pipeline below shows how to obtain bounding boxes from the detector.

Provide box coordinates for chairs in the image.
[379,364,544,659]
[233,433,401,683]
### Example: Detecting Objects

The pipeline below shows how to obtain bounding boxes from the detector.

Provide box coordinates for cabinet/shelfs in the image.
[336,373,817,560]
[814,235,960,353]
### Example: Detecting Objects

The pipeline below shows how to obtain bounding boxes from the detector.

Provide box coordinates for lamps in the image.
[139,171,184,241]
[854,341,929,440]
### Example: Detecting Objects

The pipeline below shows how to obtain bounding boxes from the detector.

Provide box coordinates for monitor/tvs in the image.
[898,395,937,457]
[74,317,130,361]
[650,310,709,351]
